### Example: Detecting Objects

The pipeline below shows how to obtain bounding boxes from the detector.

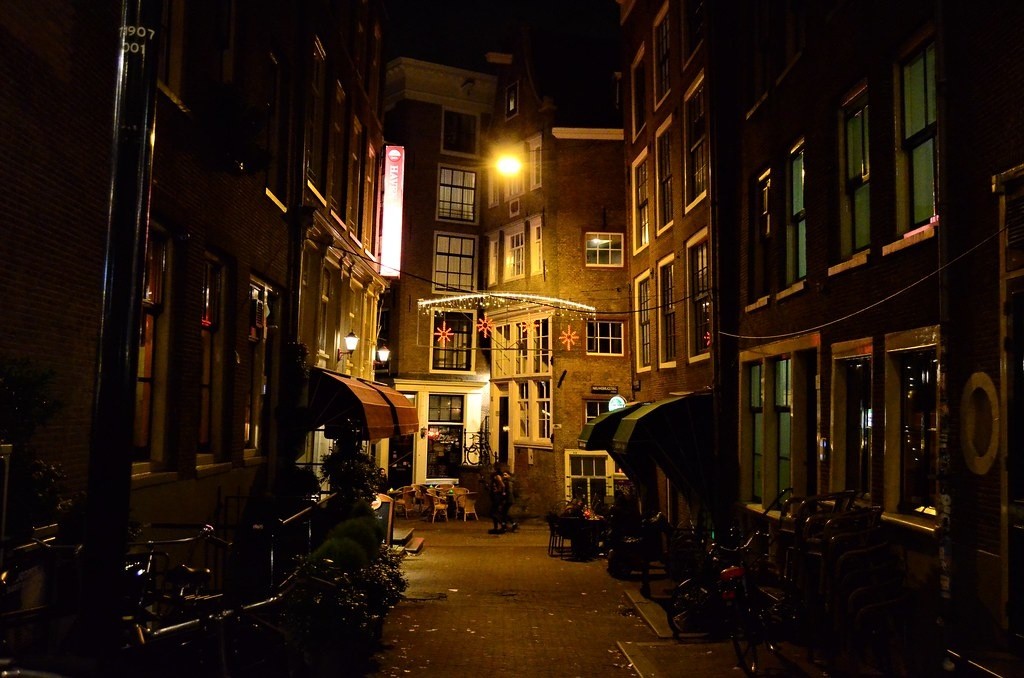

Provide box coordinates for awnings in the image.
[311,367,419,441]
[576,389,718,459]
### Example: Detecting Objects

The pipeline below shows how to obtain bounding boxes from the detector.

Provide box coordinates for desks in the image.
[446,492,477,517]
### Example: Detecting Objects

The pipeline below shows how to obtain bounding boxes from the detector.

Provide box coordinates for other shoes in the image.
[488,529,498,533]
[499,528,507,533]
[509,523,518,532]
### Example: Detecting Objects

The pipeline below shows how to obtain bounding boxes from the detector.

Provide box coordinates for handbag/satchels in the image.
[488,472,502,495]
[511,482,519,499]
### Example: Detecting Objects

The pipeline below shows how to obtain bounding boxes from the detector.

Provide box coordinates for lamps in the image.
[369,341,390,367]
[338,330,360,361]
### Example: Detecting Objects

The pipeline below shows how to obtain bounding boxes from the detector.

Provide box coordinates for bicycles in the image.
[700,527,786,676]
[669,480,945,678]
[29,493,347,678]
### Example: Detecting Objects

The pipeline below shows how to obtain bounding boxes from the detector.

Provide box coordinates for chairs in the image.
[394,486,479,523]
[545,502,650,561]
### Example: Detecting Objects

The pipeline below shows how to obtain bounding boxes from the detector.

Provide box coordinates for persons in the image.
[478,462,520,535]
[377,467,392,495]
